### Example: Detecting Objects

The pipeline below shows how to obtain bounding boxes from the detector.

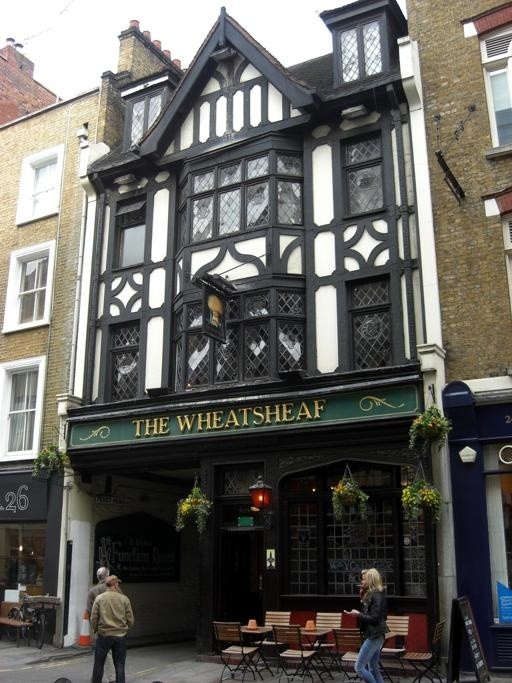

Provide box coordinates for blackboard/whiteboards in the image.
[456,596,489,681]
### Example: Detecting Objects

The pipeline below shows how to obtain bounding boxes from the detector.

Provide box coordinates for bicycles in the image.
[5,598,45,650]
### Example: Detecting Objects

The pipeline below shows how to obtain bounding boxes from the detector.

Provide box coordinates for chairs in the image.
[212,611,447,683]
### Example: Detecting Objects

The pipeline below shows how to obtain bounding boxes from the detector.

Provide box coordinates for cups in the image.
[248,620,257,628]
[306,621,314,630]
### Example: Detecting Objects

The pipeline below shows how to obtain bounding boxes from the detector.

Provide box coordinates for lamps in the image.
[249,475,275,528]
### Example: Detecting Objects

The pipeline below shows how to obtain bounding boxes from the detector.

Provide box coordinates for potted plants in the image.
[331,478,370,521]
[32,445,69,483]
[401,482,442,535]
[407,387,449,456]
[175,488,213,533]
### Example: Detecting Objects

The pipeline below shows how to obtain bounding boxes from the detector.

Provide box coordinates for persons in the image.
[84,564,123,682]
[89,574,135,682]
[342,567,393,682]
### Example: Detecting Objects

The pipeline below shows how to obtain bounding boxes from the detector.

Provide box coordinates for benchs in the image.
[0,617,34,648]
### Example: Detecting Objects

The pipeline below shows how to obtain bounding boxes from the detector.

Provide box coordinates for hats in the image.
[105,575,121,585]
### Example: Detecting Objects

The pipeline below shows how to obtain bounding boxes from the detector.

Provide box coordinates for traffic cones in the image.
[80,611,93,647]
